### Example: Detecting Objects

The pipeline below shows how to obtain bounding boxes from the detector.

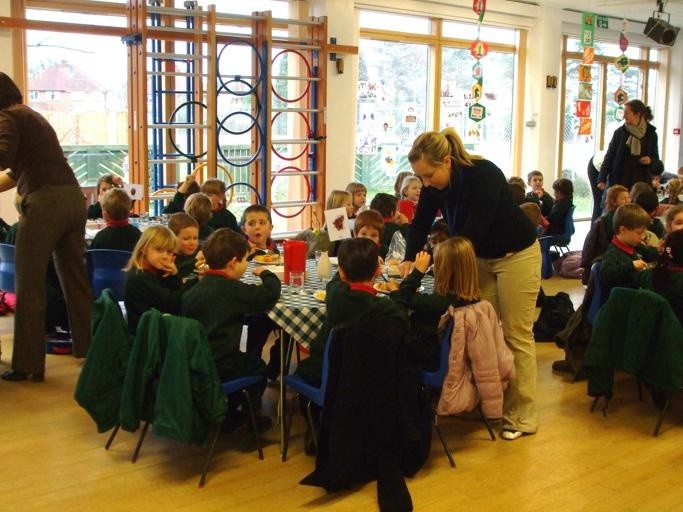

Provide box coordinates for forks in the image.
[256,248,275,256]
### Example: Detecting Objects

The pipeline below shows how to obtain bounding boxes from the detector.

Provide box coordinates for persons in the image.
[0,70,682,490]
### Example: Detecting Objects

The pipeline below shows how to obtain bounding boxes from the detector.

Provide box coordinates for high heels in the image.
[0,370,42,381]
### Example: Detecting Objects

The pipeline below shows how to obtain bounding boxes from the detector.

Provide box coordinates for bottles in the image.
[317,251,333,281]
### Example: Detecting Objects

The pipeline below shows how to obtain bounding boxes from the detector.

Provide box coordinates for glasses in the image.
[154,246,173,255]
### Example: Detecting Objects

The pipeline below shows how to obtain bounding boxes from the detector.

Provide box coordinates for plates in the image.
[373,282,426,294]
[313,291,326,302]
[252,256,284,265]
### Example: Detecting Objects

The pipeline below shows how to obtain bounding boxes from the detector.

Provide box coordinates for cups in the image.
[128,212,172,232]
[283,241,308,285]
[289,272,305,294]
[314,250,323,265]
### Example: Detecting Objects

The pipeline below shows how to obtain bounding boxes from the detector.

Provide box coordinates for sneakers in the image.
[502,429,521,439]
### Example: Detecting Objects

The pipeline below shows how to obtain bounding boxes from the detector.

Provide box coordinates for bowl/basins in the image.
[264,265,284,279]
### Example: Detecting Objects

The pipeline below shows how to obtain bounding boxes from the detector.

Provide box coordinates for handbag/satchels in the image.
[533,292,575,342]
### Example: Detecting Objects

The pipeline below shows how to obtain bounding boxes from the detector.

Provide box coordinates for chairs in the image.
[119,309,276,488]
[74,288,135,448]
[422,300,496,468]
[588,261,606,325]
[539,204,576,260]
[589,288,683,436]
[277,328,333,454]
[83,248,133,301]
[0,242,15,307]
